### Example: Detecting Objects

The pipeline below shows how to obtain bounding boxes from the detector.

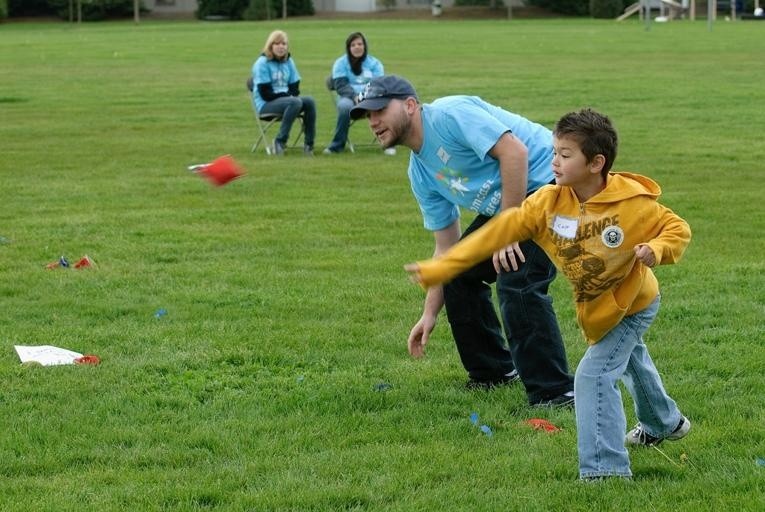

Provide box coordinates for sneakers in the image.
[324,147,331,153]
[384,147,395,155]
[304,143,314,154]
[626,415,691,447]
[510,391,574,416]
[275,142,286,154]
[459,369,521,387]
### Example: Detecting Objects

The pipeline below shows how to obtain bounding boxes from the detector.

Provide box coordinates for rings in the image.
[508,251,513,254]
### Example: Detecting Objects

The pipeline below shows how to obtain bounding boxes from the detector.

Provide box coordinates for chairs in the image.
[246,78,310,157]
[325,75,384,154]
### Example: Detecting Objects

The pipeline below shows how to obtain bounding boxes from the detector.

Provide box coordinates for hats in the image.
[349,75,419,119]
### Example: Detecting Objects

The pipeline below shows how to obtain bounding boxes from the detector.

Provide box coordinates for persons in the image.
[322,32,396,156]
[349,76,575,417]
[250,30,316,156]
[404,108,692,481]
